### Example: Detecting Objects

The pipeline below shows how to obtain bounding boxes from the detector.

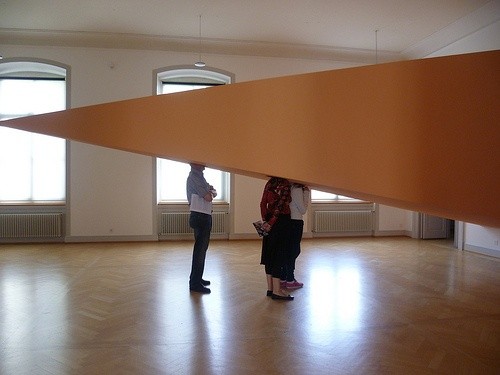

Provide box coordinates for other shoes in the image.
[272,293,295,300]
[190,285,210,293]
[266,290,272,296]
[201,279,210,286]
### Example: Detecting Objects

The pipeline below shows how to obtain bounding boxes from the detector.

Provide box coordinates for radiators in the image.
[159,211,228,236]
[312,210,374,232]
[0,212,63,237]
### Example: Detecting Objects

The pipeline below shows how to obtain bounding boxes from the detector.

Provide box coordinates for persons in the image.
[260,177,295,300]
[186,163,217,293]
[280,182,309,289]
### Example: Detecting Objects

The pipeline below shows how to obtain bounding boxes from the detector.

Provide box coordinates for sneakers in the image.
[280,282,285,286]
[285,280,303,288]
